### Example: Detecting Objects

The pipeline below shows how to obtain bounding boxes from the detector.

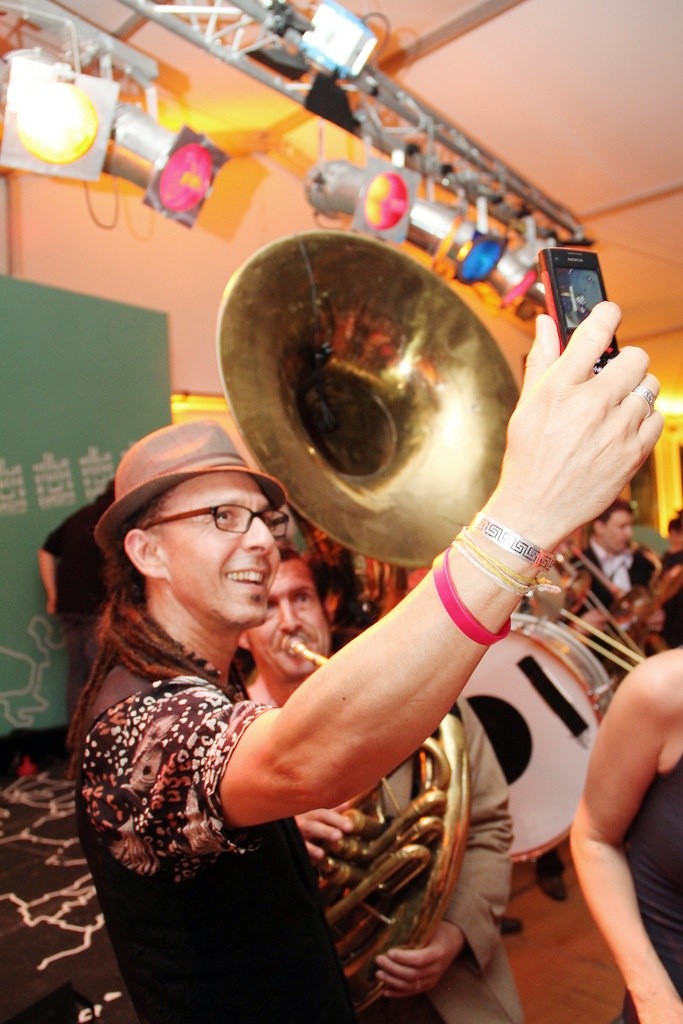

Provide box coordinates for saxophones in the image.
[604,536,682,688]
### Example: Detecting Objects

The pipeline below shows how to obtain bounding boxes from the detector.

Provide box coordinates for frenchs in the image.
[217,226,526,1014]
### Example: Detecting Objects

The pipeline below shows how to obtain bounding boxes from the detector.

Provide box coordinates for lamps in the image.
[105,39,552,312]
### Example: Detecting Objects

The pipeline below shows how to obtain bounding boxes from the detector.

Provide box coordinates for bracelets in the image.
[424,547,524,646]
[424,513,562,573]
[452,529,564,601]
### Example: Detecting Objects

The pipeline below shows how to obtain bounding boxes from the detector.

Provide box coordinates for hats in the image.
[94,419,287,552]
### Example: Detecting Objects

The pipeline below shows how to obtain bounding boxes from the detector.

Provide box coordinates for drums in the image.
[458,613,616,865]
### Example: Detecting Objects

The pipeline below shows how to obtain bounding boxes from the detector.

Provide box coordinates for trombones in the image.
[554,539,647,667]
[518,592,644,672]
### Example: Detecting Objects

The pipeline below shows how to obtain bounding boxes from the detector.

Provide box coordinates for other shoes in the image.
[539,870,568,901]
[501,917,521,934]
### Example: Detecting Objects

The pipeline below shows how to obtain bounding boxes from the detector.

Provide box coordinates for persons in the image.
[36,480,127,768]
[550,500,666,646]
[481,848,571,943]
[198,538,523,1024]
[654,520,682,649]
[70,298,682,1023]
[569,583,682,1024]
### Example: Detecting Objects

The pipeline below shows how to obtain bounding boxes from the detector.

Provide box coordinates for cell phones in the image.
[537,247,621,379]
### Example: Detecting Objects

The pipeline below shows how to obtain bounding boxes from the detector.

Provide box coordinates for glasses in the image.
[142,503,291,536]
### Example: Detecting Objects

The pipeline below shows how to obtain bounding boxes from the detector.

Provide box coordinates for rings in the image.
[629,384,656,419]
